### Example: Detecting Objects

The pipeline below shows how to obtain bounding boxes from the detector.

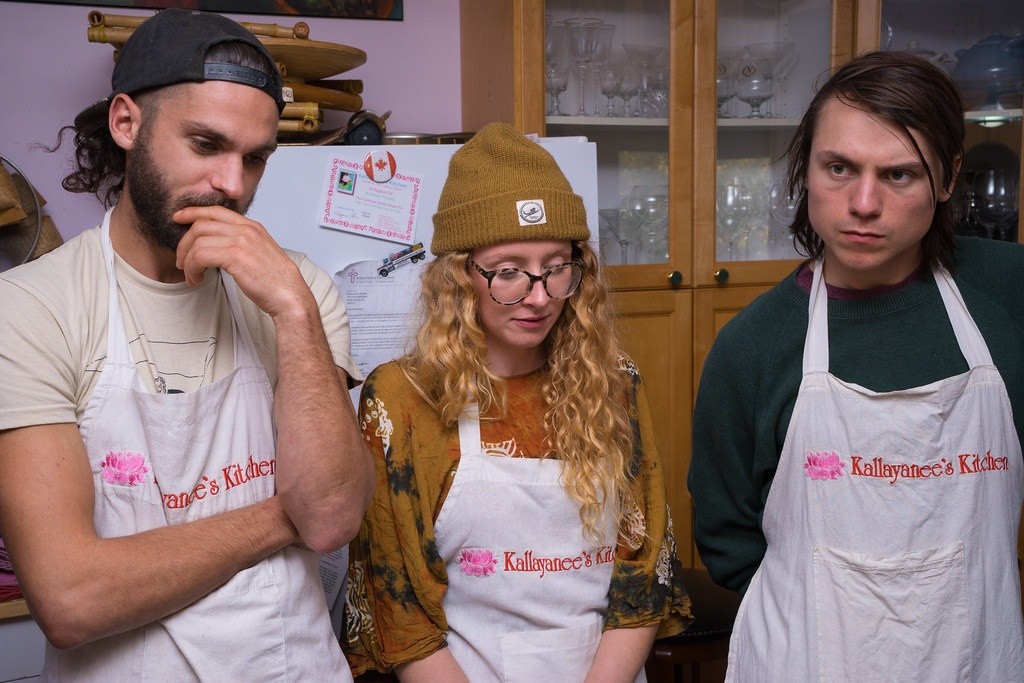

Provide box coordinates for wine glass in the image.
[943,165,1021,243]
[598,181,670,265]
[541,11,668,116]
[714,40,798,118]
[714,176,809,259]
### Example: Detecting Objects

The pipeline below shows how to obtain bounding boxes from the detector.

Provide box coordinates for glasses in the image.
[466,257,587,306]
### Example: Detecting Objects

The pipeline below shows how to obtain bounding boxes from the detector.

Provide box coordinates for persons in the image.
[685,54,1024,683]
[1,6,379,683]
[338,124,696,683]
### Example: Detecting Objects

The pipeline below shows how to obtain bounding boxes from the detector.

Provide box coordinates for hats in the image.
[73,7,288,141]
[430,122,592,256]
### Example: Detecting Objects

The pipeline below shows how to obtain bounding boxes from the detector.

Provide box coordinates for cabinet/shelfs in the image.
[510,0,1024,626]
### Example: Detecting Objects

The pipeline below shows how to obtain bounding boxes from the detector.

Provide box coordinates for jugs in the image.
[894,40,951,81]
[952,30,1023,110]
